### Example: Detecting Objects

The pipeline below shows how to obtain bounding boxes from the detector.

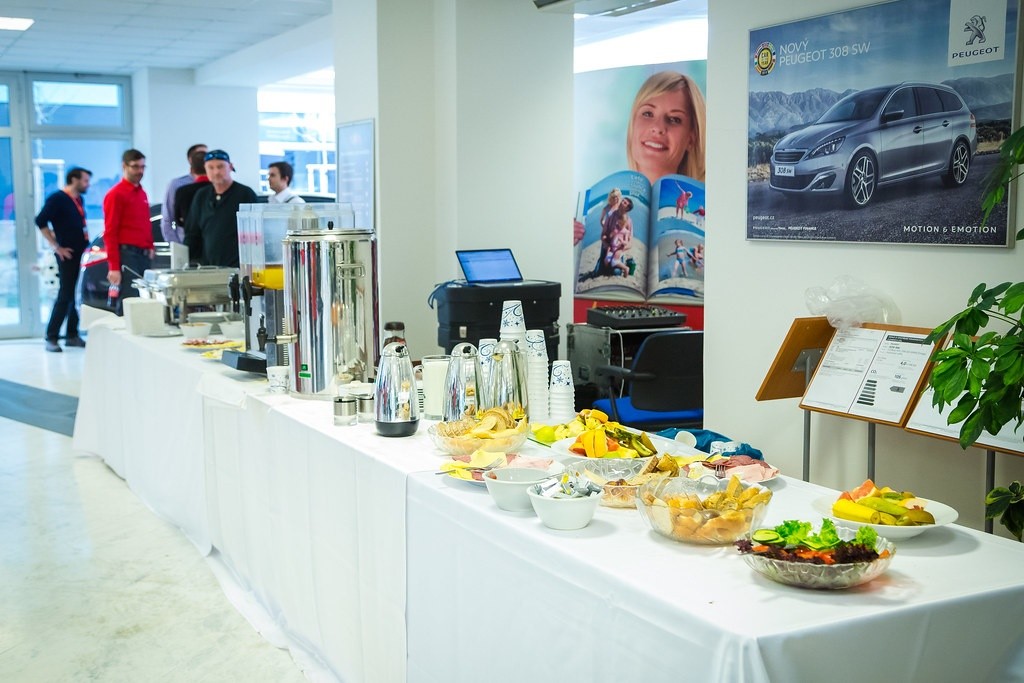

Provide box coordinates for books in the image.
[573,170,704,307]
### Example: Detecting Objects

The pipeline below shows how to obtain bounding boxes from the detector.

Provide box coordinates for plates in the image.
[551,437,676,461]
[182,346,241,352]
[198,349,231,361]
[188,312,231,334]
[143,330,181,336]
[690,460,781,483]
[442,454,567,486]
[826,496,959,540]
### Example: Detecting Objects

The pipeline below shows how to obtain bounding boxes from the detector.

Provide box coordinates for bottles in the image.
[383,322,407,351]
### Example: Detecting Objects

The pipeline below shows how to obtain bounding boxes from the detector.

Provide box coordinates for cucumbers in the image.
[752,529,787,547]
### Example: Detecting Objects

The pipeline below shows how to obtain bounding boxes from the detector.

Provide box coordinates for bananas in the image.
[532,409,609,442]
[833,499,897,525]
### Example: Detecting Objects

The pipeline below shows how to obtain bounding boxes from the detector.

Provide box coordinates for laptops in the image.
[456,249,546,287]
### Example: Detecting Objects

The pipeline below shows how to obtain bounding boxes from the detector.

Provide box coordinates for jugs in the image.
[376,343,419,436]
[444,338,531,425]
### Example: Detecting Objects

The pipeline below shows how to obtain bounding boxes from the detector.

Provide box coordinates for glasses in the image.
[125,163,145,169]
[204,154,228,162]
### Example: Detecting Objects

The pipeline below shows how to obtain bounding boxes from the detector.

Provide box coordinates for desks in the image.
[567,321,691,387]
[70,319,1024,683]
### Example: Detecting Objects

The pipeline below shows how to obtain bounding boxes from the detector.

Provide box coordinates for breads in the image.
[442,407,529,457]
[638,452,681,480]
[643,477,771,545]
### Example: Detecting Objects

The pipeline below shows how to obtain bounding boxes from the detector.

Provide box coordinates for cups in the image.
[413,355,451,420]
[500,300,576,428]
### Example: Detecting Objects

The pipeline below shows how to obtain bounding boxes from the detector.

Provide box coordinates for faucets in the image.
[226,271,264,316]
[247,320,299,361]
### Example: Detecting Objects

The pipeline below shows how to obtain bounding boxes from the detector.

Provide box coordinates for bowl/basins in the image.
[218,321,244,339]
[564,459,645,509]
[741,526,896,589]
[428,423,531,456]
[527,483,604,530]
[635,474,773,545]
[180,323,213,338]
[481,467,549,512]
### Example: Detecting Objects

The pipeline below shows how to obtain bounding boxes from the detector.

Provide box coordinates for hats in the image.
[206,150,236,171]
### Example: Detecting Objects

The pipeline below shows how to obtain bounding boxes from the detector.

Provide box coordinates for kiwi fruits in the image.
[630,431,658,457]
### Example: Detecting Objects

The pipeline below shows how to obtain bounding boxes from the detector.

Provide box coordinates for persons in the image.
[35,167,92,352]
[175,151,210,227]
[572,70,707,322]
[102,150,155,317]
[183,151,258,267]
[160,144,207,245]
[268,162,305,202]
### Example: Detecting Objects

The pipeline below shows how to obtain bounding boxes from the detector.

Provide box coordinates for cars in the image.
[79,193,335,325]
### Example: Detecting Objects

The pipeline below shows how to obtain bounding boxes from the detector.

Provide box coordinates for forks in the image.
[715,465,726,481]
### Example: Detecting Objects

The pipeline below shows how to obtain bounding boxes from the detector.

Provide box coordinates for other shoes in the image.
[46,340,62,352]
[65,337,86,347]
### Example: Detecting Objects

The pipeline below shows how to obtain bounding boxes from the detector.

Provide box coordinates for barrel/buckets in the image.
[283,229,378,394]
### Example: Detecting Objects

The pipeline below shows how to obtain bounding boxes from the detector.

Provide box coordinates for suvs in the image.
[770,80,978,211]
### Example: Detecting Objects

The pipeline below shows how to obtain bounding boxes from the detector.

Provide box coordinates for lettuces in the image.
[768,517,878,552]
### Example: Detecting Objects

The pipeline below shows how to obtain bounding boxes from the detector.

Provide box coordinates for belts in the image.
[119,244,150,255]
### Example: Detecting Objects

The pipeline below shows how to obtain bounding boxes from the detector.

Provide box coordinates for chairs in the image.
[592,330,704,432]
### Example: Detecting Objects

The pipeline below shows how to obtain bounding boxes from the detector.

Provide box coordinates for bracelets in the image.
[53,244,59,250]
